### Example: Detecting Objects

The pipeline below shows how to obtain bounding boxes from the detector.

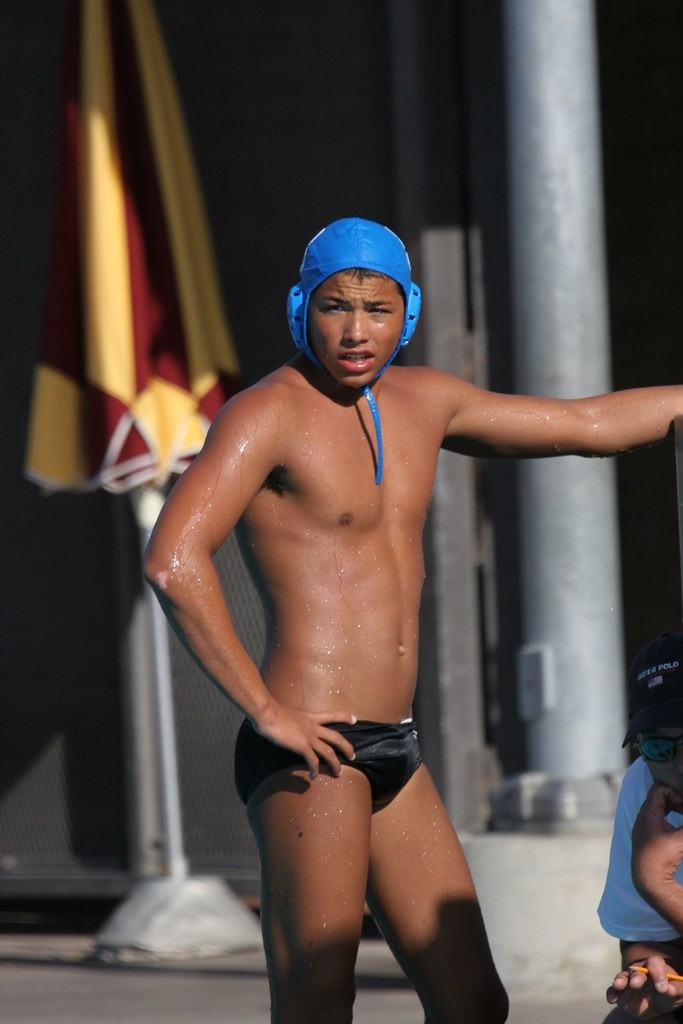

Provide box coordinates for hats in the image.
[622,630,683,748]
[287,217,422,389]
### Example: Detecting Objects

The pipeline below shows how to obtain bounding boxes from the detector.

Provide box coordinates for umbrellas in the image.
[21,0,242,878]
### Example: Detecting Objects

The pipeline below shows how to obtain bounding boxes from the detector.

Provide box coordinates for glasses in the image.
[634,735,683,763]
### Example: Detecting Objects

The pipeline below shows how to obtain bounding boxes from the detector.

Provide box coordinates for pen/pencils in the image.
[627,964,683,982]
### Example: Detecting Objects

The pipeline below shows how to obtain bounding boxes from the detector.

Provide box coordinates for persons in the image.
[140,217,683,1024]
[597,627,683,1024]
